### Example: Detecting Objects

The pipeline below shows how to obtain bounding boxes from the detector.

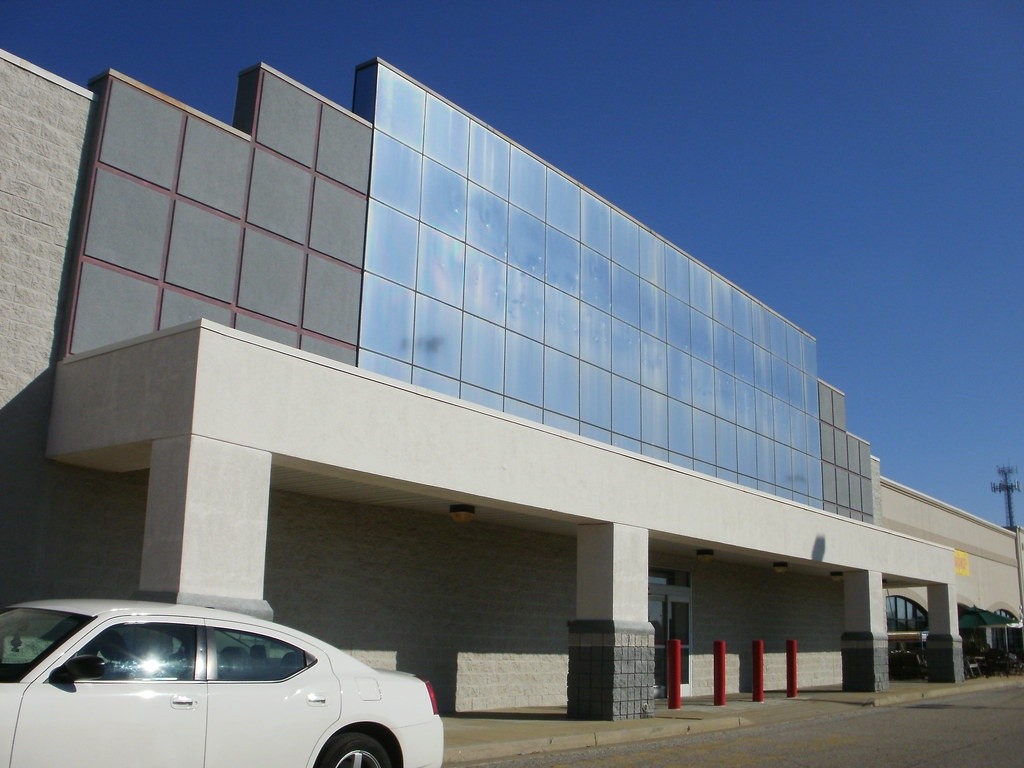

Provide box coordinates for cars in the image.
[0,596,445,768]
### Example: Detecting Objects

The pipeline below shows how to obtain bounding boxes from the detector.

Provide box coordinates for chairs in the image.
[219,645,260,681]
[985,649,1018,679]
[77,630,130,679]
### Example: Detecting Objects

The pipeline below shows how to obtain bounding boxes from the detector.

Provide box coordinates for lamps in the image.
[449,503,475,524]
[773,560,789,574]
[695,548,715,562]
[830,571,844,581]
